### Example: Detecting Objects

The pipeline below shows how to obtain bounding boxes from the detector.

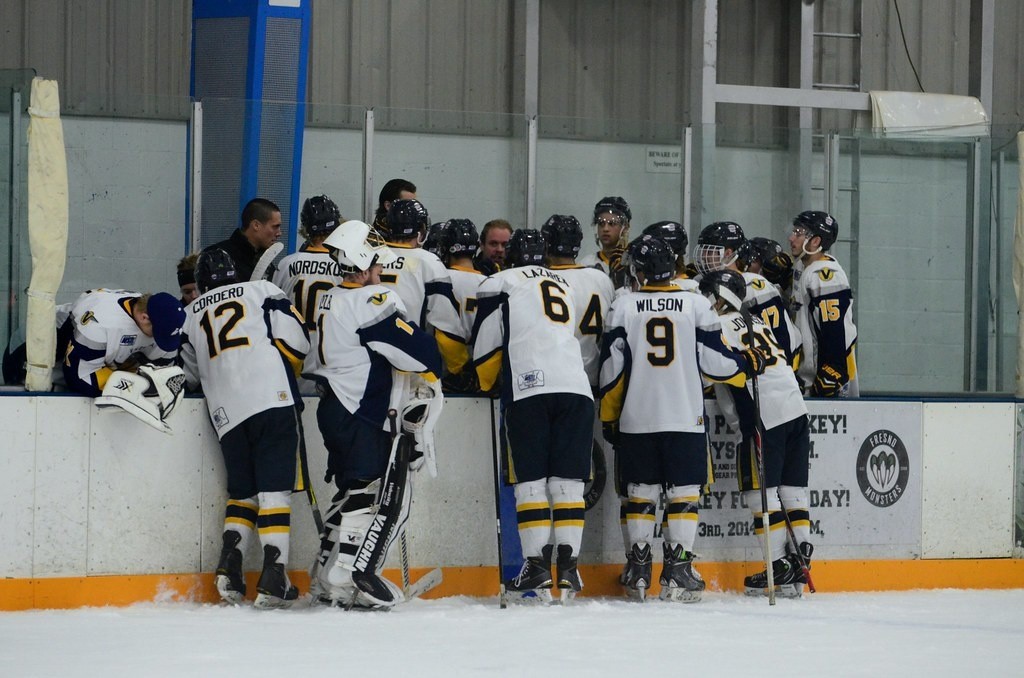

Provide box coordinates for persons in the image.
[177,179,861,614]
[3,289,190,432]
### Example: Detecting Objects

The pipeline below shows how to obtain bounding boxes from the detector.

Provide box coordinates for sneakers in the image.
[659,542,706,603]
[557,544,584,602]
[254,544,298,609]
[215,530,247,605]
[619,543,653,602]
[744,542,814,597]
[505,544,553,604]
[310,512,406,611]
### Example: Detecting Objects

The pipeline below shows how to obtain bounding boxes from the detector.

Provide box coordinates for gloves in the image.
[811,375,842,398]
[602,419,625,449]
[738,345,766,379]
[453,364,478,392]
[796,375,806,394]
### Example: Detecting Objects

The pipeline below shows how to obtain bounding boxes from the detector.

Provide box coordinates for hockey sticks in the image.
[386,409,443,602]
[490,396,507,609]
[248,242,331,551]
[777,490,816,594]
[717,284,776,606]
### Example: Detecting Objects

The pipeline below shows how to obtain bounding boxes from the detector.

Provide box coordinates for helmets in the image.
[387,199,432,246]
[301,194,341,235]
[693,210,838,311]
[624,221,688,281]
[594,197,632,222]
[322,221,398,273]
[504,214,583,267]
[194,248,239,293]
[422,218,480,260]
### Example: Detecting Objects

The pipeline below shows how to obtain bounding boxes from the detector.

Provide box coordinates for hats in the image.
[147,292,187,352]
